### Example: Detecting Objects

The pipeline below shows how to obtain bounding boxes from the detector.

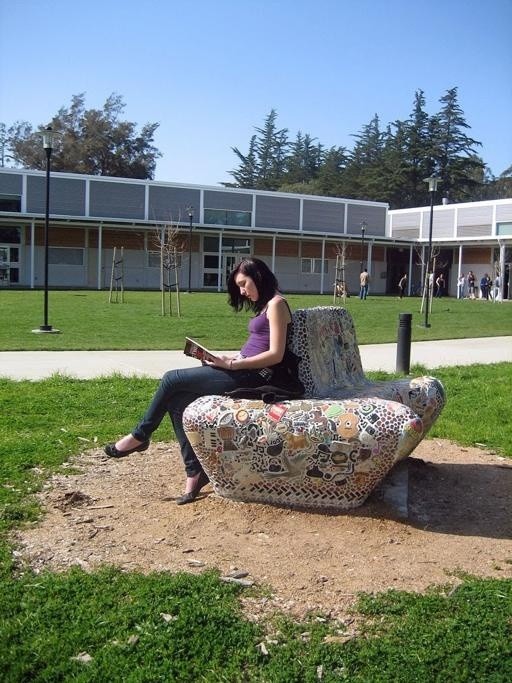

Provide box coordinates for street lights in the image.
[35,127,63,330]
[185,206,196,293]
[423,173,444,327]
[360,221,368,273]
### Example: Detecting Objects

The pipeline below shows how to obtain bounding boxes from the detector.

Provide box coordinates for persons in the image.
[457,271,500,300]
[434,273,445,299]
[359,268,369,300]
[397,273,408,299]
[103,258,293,506]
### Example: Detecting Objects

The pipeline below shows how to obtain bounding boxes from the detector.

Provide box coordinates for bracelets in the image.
[228,359,235,372]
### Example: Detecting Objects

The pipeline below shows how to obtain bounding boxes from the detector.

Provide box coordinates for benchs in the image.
[181,304,446,515]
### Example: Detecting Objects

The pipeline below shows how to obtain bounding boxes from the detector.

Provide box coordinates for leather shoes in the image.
[175,470,210,505]
[103,438,151,459]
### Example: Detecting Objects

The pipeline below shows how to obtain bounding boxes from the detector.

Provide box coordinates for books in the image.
[183,337,219,361]
